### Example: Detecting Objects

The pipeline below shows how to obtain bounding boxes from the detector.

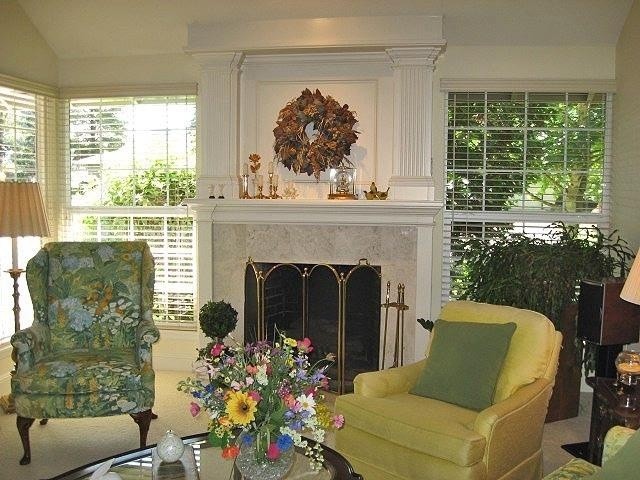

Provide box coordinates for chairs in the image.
[332,299,563,479]
[11,241,160,466]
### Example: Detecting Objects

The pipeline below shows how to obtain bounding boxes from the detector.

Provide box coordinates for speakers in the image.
[576,278,639,346]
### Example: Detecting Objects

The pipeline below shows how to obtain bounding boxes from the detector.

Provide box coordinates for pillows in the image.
[408,318,517,413]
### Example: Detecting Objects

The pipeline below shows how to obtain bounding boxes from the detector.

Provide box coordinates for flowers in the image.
[175,322,345,476]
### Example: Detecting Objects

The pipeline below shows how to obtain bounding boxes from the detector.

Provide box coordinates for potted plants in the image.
[449,220,636,423]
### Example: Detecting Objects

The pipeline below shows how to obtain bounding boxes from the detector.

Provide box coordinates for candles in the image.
[242,161,280,187]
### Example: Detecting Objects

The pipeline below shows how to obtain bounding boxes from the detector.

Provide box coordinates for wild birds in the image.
[363,182,390,200]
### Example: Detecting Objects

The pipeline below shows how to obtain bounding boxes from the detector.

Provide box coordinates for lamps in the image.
[0,180,52,376]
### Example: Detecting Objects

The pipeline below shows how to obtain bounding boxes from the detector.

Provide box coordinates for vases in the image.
[235,433,296,480]
